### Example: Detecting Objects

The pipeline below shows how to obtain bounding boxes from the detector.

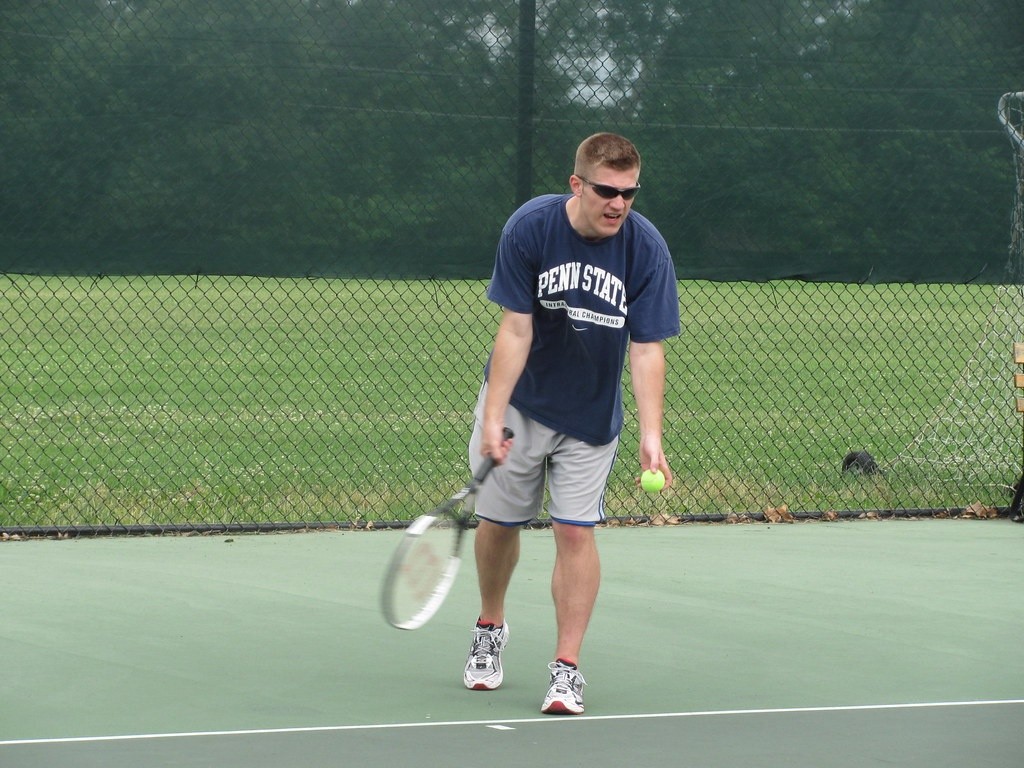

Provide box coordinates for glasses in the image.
[576,175,641,201]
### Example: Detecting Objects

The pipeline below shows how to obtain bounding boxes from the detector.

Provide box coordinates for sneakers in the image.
[541,657,585,714]
[464,617,510,690]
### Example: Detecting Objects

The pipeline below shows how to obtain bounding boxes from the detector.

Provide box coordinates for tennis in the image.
[640,468,666,494]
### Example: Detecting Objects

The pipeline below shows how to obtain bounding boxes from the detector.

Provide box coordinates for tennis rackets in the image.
[381,426,514,631]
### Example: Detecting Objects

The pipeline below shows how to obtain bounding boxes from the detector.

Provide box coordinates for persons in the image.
[462,132,683,715]
